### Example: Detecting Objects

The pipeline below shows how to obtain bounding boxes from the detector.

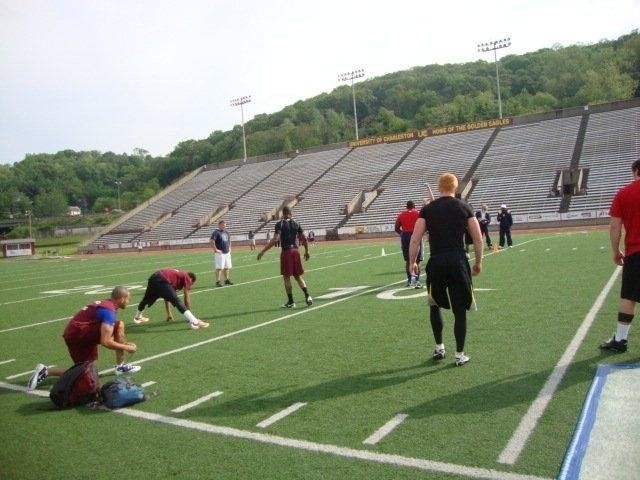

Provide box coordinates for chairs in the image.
[423,107,638,222]
[89,136,423,237]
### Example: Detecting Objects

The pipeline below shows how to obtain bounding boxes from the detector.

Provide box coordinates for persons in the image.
[137,236,144,256]
[243,200,514,309]
[408,172,486,368]
[27,283,137,390]
[209,219,235,289]
[598,158,640,356]
[133,266,209,329]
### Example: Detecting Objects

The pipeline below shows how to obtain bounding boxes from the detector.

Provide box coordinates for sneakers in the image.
[190,319,209,329]
[601,336,628,353]
[115,363,142,376]
[415,281,422,288]
[28,364,47,391]
[455,355,470,365]
[281,302,296,308]
[134,316,150,324]
[434,349,445,359]
[306,296,313,306]
[216,281,224,287]
[225,279,233,285]
[408,281,413,287]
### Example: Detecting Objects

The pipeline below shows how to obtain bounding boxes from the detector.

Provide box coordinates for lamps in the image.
[227,94,249,104]
[337,70,366,81]
[476,35,513,52]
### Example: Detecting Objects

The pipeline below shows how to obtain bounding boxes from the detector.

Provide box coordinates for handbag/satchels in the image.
[50,361,99,409]
[101,379,146,409]
